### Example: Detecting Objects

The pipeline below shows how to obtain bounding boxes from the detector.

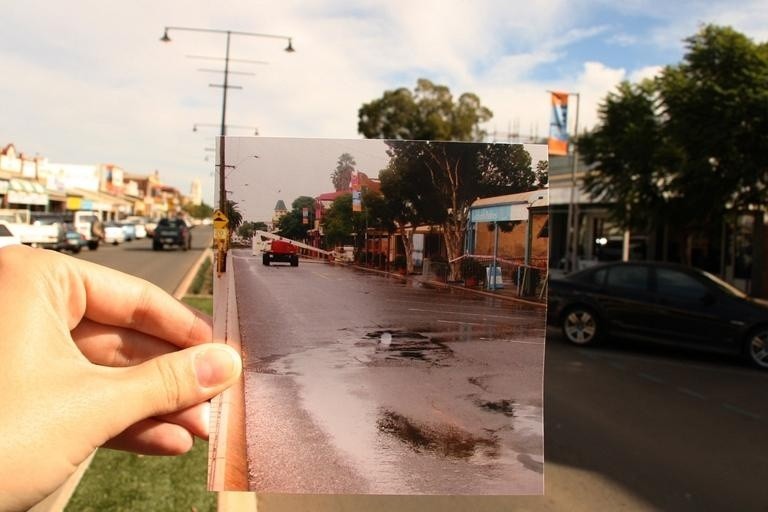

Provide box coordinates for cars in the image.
[0,209,147,254]
[547,259,768,372]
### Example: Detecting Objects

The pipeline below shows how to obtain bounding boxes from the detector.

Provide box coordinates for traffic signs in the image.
[220,155,258,215]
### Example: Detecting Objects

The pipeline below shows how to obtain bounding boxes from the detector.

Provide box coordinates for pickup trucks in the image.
[152,218,193,251]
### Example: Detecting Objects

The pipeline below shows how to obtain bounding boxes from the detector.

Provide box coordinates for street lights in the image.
[161,25,297,133]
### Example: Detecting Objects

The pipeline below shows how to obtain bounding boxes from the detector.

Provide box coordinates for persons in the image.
[1,242,242,511]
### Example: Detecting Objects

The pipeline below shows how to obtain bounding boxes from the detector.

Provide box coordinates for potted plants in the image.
[396,255,408,275]
[459,257,482,287]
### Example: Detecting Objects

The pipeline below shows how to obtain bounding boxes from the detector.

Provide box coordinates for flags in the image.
[303,208,308,224]
[352,183,362,211]
[547,93,568,156]
